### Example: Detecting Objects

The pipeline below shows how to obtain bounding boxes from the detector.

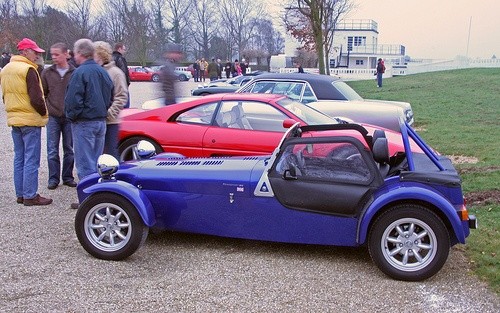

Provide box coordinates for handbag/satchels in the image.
[221,67,227,77]
[204,70,208,77]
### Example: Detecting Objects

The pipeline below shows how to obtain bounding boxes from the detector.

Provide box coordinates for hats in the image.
[242,58,245,61]
[17,38,46,53]
[235,59,238,62]
[217,58,221,62]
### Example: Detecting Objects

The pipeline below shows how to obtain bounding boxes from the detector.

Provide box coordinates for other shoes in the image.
[377,85,382,87]
[71,203,80,209]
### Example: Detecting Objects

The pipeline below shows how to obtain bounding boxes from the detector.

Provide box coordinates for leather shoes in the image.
[48,183,58,189]
[63,181,77,187]
[17,197,23,203]
[23,194,53,206]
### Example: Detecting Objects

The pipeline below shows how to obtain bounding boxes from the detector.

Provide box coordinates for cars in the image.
[141,73,415,134]
[71,115,478,283]
[105,92,441,165]
[190,72,314,103]
[151,66,193,81]
[128,65,161,82]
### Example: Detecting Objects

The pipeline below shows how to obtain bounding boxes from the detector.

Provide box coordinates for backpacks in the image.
[381,60,385,73]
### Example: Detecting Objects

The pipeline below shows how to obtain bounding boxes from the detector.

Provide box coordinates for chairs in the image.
[232,105,253,129]
[222,111,242,128]
[372,129,390,176]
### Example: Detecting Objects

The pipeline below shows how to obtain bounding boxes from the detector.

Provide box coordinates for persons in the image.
[0,52,248,85]
[296,63,304,73]
[91,41,128,162]
[64,39,113,210]
[0,38,52,206]
[42,43,78,190]
[111,43,130,108]
[158,47,184,106]
[377,58,386,87]
